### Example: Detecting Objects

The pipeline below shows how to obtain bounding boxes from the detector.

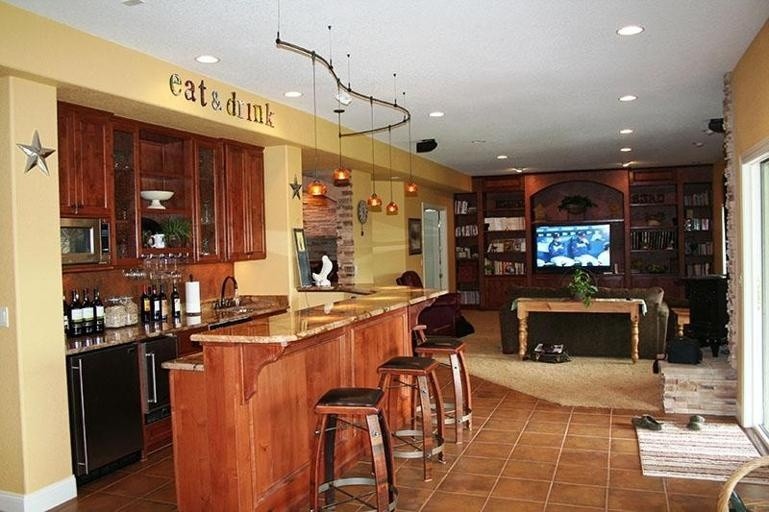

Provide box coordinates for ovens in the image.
[59,218,109,265]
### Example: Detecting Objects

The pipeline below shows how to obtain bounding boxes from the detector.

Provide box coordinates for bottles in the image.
[63,289,70,338]
[72,289,83,336]
[93,289,105,333]
[158,285,167,320]
[141,284,150,323]
[171,279,180,318]
[151,284,160,321]
[147,286,152,296]
[83,289,93,335]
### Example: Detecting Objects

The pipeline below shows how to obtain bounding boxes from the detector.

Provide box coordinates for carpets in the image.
[423,305,739,411]
[635,418,769,484]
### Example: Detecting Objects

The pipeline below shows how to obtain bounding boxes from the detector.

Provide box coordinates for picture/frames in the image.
[407,217,423,254]
[293,229,313,288]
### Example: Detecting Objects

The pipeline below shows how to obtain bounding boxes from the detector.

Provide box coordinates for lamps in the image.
[308,53,327,206]
[333,79,350,187]
[405,117,417,198]
[386,127,398,215]
[368,99,382,212]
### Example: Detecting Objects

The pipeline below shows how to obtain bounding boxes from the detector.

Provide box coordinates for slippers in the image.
[688,415,707,431]
[631,414,663,431]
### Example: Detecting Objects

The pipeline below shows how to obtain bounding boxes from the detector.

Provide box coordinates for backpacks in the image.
[653,335,702,374]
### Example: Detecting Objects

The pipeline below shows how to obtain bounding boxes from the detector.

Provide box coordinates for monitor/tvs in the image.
[531,224,613,275]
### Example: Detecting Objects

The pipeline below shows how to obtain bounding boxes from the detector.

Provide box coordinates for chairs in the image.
[376,356,448,482]
[308,388,400,512]
[410,338,473,446]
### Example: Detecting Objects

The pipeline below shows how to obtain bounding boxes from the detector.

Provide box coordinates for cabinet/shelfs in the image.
[142,334,181,459]
[69,344,145,485]
[113,114,226,266]
[678,165,714,274]
[224,140,266,262]
[455,192,480,308]
[629,167,681,299]
[177,326,210,358]
[478,177,528,309]
[58,99,113,220]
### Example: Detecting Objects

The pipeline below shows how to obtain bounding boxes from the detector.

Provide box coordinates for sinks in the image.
[217,306,264,313]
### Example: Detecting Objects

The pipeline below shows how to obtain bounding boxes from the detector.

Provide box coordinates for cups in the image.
[149,233,165,249]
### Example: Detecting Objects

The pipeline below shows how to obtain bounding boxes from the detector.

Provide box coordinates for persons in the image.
[537,231,610,265]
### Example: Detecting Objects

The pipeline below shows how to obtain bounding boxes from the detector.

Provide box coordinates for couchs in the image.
[682,274,731,355]
[396,269,464,337]
[500,286,670,360]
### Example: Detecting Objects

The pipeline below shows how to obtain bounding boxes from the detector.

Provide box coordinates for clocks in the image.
[358,199,368,237]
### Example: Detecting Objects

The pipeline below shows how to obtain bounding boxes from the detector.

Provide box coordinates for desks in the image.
[517,297,639,365]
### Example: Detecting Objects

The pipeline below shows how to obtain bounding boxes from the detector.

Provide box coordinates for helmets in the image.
[578,231,585,237]
[553,233,559,238]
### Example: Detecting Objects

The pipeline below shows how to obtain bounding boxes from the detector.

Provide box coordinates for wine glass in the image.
[123,252,183,280]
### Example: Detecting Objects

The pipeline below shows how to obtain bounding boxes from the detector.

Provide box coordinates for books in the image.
[698,241,712,255]
[457,226,479,236]
[693,218,708,230]
[685,191,708,206]
[485,257,525,275]
[484,217,526,231]
[687,262,710,275]
[457,247,472,260]
[454,200,468,215]
[487,238,526,252]
[456,290,479,305]
[631,229,676,249]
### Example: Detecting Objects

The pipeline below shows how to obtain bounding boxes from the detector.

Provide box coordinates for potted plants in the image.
[162,215,190,250]
[568,264,595,302]
[558,195,595,214]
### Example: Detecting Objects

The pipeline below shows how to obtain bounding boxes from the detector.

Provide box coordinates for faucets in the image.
[221,276,238,308]
